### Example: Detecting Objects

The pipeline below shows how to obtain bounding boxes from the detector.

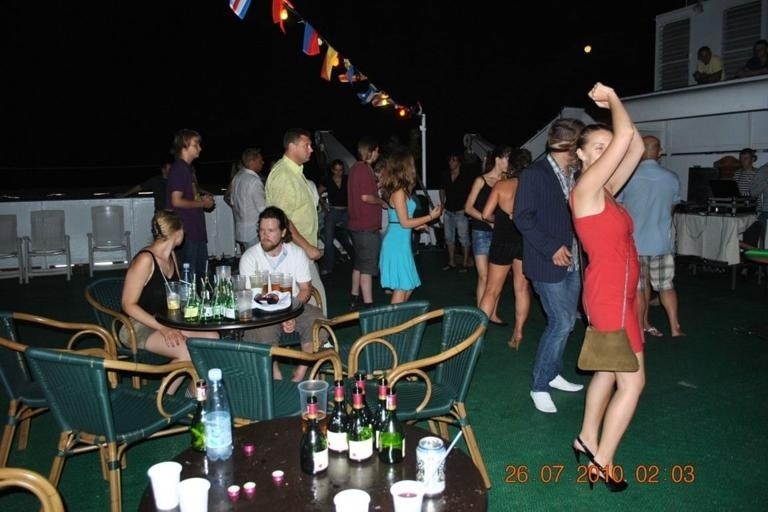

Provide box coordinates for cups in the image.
[297,380,329,439]
[177,478,210,512]
[250,269,293,297]
[333,489,370,512]
[232,275,246,292]
[390,480,424,512]
[236,289,252,322]
[165,282,181,319]
[146,461,182,510]
[215,266,231,289]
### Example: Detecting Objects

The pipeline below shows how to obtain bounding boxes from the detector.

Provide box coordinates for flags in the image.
[228,0,392,108]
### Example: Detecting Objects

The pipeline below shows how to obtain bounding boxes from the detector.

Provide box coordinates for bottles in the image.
[206,367,232,461]
[348,374,407,465]
[191,379,206,452]
[180,277,236,326]
[300,397,329,474]
[178,263,192,314]
[329,380,348,454]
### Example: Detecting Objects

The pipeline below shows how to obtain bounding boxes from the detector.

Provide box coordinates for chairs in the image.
[87,205,131,278]
[314,303,436,437]
[0,468,64,512]
[0,215,23,285]
[189,339,341,429]
[733,242,768,289]
[25,348,199,512]
[278,286,324,343]
[350,307,490,488]
[87,277,171,388]
[24,210,71,283]
[0,312,116,470]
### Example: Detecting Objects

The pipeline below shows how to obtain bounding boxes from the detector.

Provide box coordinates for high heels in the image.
[586,458,629,492]
[572,436,594,464]
[508,336,523,352]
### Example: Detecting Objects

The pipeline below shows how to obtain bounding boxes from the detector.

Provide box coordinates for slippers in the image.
[459,267,473,273]
[644,326,663,337]
[442,263,456,270]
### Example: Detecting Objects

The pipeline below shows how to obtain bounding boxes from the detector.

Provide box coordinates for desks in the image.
[674,214,760,287]
[139,415,489,511]
[155,286,305,433]
[0,193,242,266]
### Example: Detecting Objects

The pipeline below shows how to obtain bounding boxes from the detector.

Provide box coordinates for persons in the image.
[751,162,768,281]
[569,82,645,491]
[514,118,586,413]
[318,140,442,309]
[735,39,768,78]
[226,134,325,383]
[121,212,219,395]
[158,128,215,282]
[615,136,686,343]
[693,45,726,84]
[732,148,763,276]
[439,148,532,350]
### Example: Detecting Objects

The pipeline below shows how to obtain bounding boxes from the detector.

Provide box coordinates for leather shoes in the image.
[548,374,585,393]
[529,391,557,414]
[489,320,508,327]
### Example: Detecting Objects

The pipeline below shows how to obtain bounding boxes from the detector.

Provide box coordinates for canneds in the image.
[416,436,446,499]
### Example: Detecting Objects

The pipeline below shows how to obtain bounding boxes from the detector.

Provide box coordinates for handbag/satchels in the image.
[577,326,639,372]
